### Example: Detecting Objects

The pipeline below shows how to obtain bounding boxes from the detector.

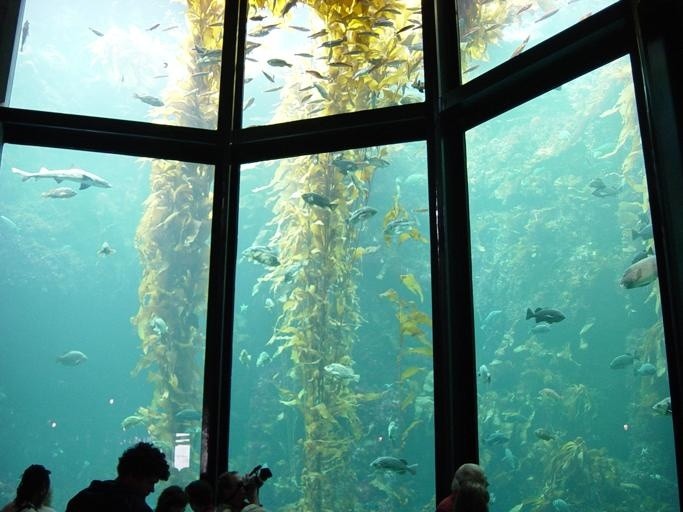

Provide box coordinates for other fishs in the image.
[117,0,683,511]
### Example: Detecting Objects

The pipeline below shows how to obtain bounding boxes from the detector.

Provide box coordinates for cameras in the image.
[239,468,272,491]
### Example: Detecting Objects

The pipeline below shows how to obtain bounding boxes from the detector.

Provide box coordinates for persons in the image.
[0,462,58,511]
[430,462,491,512]
[153,486,188,511]
[184,478,230,511]
[216,470,267,511]
[62,440,169,511]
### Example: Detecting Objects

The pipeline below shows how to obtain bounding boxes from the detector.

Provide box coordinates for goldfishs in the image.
[9,166,113,191]
